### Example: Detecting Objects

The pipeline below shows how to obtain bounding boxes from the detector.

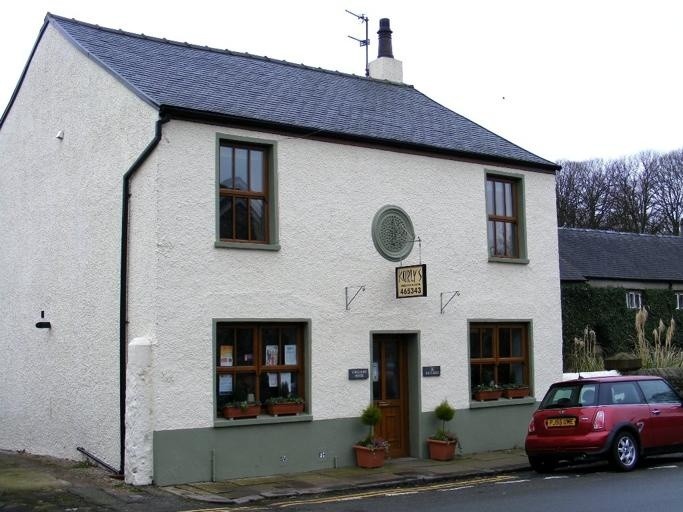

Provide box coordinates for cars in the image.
[524,371,683,471]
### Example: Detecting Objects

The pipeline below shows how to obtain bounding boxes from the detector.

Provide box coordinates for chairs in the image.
[581,390,595,405]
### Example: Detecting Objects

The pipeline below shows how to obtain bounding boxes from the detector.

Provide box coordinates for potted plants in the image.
[424,398,458,462]
[264,397,305,417]
[473,380,504,402]
[503,383,530,400]
[222,401,262,421]
[351,404,390,469]
